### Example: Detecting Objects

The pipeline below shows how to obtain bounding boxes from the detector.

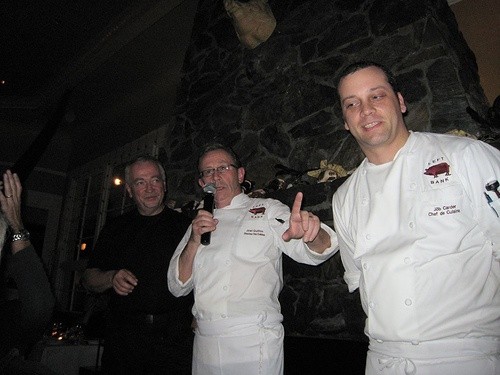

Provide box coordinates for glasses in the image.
[200,164,238,177]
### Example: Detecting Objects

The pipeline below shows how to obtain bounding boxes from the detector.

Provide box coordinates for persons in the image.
[168,146,340,375]
[0,169,56,375]
[332,59,500,375]
[83,154,193,375]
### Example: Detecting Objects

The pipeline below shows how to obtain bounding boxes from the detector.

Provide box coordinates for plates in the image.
[48,342,62,345]
[88,340,99,344]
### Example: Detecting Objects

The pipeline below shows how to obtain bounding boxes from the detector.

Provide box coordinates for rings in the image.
[6,195,13,197]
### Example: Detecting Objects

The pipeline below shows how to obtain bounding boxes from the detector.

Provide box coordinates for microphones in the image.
[200,182,216,245]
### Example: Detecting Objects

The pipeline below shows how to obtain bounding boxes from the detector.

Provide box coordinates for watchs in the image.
[11,229,31,242]
